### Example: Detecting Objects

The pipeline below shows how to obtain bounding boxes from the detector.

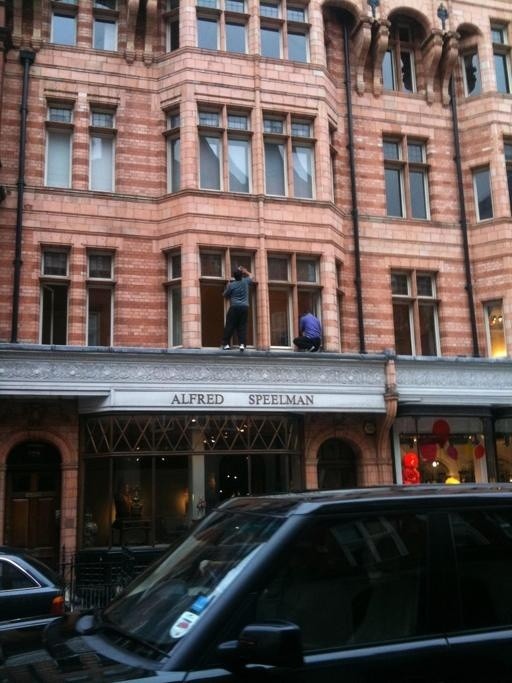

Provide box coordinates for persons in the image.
[223,266,255,351]
[199,558,236,577]
[293,308,322,353]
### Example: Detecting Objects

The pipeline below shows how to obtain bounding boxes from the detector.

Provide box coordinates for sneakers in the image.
[240,344,246,351]
[306,346,321,354]
[222,344,231,349]
